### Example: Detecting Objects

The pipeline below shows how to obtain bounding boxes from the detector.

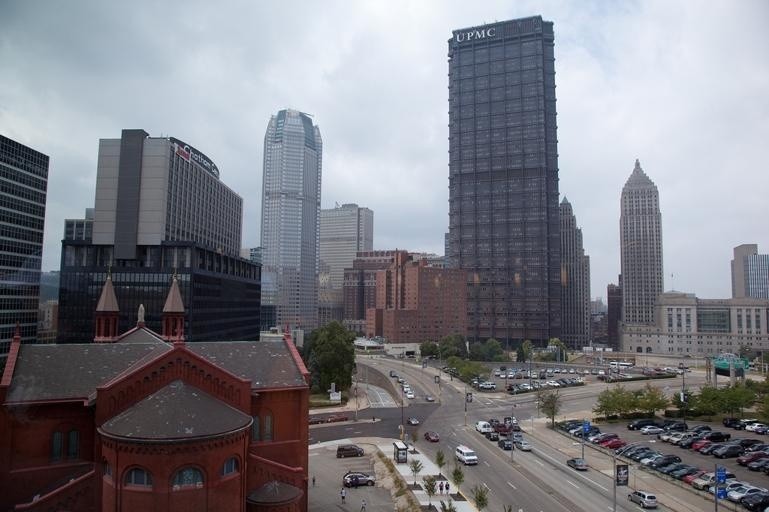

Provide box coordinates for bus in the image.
[610,361,633,370]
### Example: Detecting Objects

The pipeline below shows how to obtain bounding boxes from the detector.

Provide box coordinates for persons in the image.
[347,475,352,488]
[439,481,444,494]
[340,487,347,504]
[360,499,366,512]
[373,416,375,424]
[445,482,449,495]
[355,474,359,488]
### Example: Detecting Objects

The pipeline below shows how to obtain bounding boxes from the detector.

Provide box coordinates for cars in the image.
[494,364,606,378]
[407,416,419,425]
[558,419,768,511]
[443,365,497,390]
[612,373,631,379]
[309,415,348,425]
[391,370,415,399]
[424,431,440,442]
[426,396,434,401]
[475,416,531,451]
[507,377,584,390]
[644,363,691,376]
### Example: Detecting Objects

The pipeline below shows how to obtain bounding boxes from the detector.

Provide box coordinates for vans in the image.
[337,444,363,458]
[456,445,478,465]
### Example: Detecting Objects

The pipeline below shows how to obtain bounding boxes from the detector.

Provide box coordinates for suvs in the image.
[343,472,375,487]
